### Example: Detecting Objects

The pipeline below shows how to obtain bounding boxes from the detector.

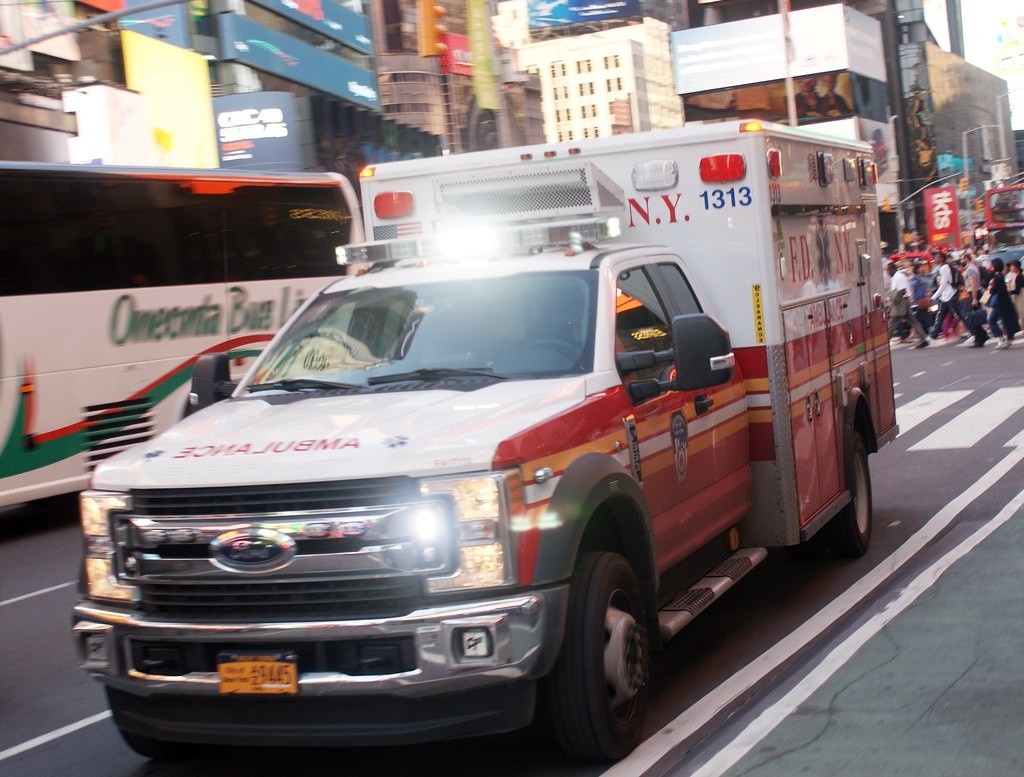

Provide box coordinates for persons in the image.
[879,240,1024,349]
[391,299,488,370]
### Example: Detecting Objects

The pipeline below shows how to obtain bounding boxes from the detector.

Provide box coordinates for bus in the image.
[0,158,366,508]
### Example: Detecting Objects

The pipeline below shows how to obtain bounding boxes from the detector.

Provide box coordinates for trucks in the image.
[70,119,899,762]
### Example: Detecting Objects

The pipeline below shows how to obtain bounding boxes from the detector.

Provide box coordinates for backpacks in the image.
[941,263,962,288]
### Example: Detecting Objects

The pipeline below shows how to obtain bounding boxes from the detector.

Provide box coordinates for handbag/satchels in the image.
[917,296,933,310]
[965,305,988,328]
[979,285,998,307]
[887,289,902,305]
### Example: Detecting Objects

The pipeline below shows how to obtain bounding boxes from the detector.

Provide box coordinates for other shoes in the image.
[915,340,929,349]
[968,343,984,348]
[994,339,1012,349]
